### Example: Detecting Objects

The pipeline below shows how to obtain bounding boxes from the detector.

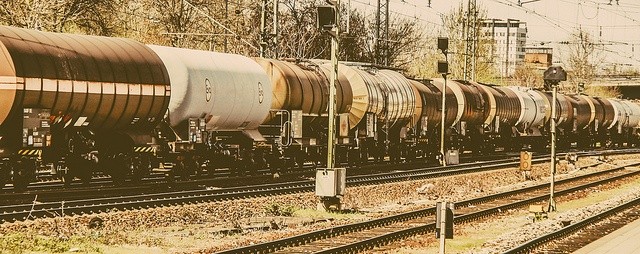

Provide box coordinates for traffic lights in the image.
[317,6,337,28]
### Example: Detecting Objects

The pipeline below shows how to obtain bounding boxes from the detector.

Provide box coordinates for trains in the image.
[0,26,640,193]
